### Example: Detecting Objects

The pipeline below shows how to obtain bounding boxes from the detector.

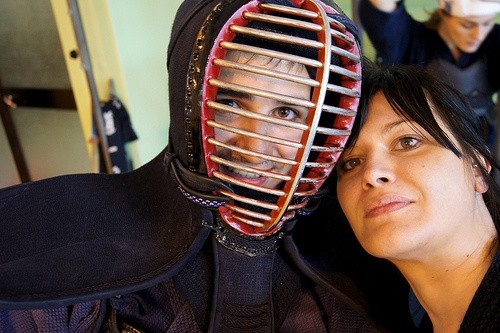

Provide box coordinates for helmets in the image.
[167,1,368,255]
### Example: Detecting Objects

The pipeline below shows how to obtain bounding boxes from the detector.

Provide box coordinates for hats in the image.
[438,0,500,18]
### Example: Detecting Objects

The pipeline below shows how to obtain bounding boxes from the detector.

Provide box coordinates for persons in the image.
[336,57,500,333]
[359,0,500,152]
[0,0,404,333]
